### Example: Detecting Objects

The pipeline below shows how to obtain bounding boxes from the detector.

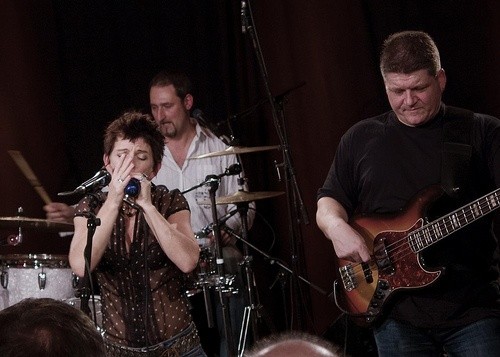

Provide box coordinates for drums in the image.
[187,237,215,278]
[66,294,102,327]
[0,254,77,310]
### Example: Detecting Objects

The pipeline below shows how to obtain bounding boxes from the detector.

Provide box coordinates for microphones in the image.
[193,109,229,147]
[242,0,246,32]
[197,210,238,239]
[75,170,111,194]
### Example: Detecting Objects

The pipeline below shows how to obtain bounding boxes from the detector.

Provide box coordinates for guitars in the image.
[333,182,500,329]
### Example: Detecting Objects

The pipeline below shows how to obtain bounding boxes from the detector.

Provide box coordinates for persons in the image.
[44,68,257,357]
[316,31,500,357]
[67,110,208,357]
[0,298,106,357]
[240,331,344,357]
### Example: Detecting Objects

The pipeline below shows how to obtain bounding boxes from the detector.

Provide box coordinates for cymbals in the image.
[0,216,74,234]
[197,191,287,205]
[188,145,280,159]
